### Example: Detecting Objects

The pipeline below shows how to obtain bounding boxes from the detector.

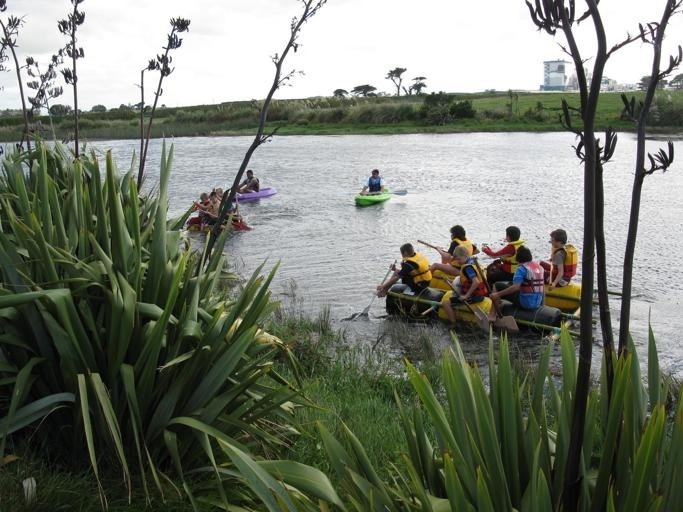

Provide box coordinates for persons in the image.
[189,192,209,225]
[218,189,241,225]
[235,169,259,193]
[480,225,528,290]
[539,228,578,290]
[375,242,433,299]
[213,186,224,202]
[192,191,222,225]
[441,243,489,330]
[360,168,385,195]
[428,224,480,278]
[488,244,544,311]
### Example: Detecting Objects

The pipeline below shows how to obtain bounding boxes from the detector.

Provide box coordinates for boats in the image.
[230,186,278,201]
[187,217,247,232]
[354,187,392,206]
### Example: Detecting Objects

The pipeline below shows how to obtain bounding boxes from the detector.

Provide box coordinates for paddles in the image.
[364,261,398,313]
[203,212,251,231]
[444,280,490,332]
[360,189,407,196]
[474,259,518,330]
